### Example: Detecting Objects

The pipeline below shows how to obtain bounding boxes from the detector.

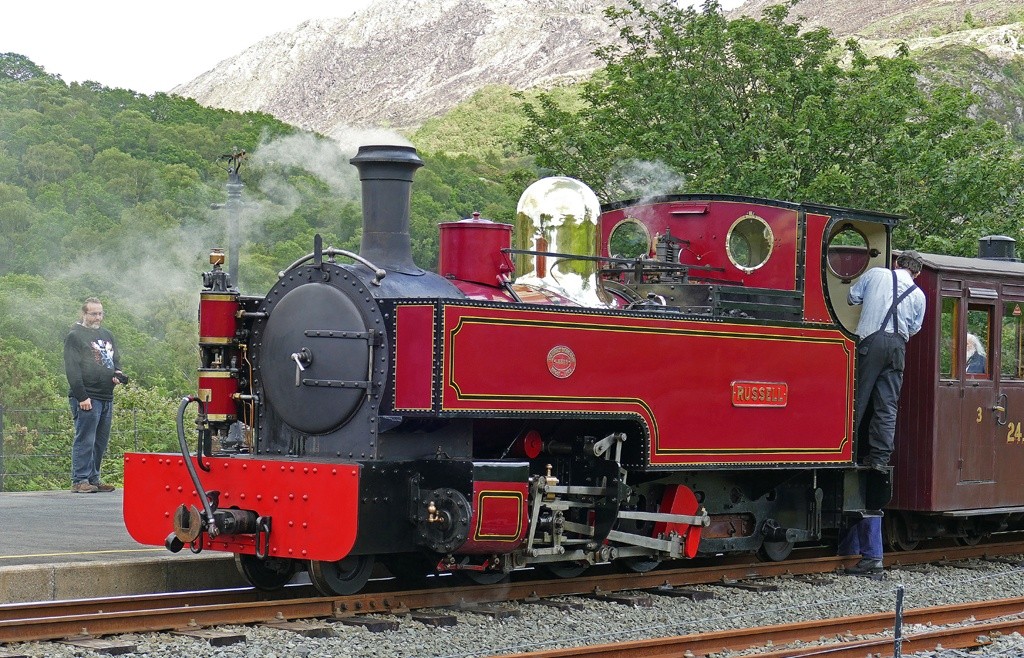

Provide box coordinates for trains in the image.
[123,146,1024,599]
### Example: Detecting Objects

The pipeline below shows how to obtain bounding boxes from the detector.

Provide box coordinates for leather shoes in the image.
[845,560,884,575]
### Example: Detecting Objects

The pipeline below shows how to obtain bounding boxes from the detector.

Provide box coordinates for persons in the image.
[847,250,926,473]
[63,298,122,493]
[838,511,883,576]
[966,332,986,373]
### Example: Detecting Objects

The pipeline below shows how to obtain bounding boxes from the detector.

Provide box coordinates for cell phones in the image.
[114,373,128,384]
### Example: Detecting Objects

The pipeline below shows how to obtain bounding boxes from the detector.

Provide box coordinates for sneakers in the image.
[71,482,115,493]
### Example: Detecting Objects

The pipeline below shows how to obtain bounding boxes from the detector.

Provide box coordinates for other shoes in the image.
[863,455,885,472]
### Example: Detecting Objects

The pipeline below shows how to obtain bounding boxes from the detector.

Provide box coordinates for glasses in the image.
[88,312,102,316]
